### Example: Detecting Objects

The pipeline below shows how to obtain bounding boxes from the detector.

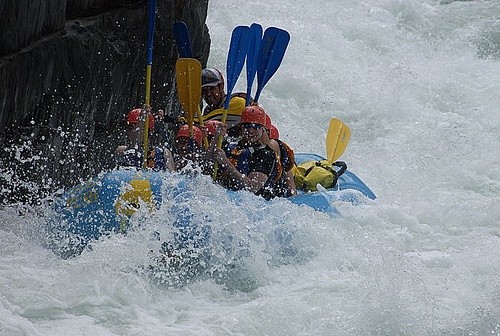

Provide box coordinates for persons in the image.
[115,67,297,201]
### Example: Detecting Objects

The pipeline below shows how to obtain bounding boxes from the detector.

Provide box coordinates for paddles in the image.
[326,117,351,169]
[175,59,202,174]
[142,0,158,170]
[245,22,263,107]
[214,25,249,180]
[253,27,290,106]
[173,21,210,152]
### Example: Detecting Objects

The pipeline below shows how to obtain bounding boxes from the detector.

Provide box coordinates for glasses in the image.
[240,122,262,130]
[202,85,220,91]
[178,137,199,148]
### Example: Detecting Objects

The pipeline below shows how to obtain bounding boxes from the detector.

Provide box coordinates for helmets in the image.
[175,125,202,146]
[199,66,224,88]
[206,120,222,135]
[270,125,279,140]
[127,108,155,129]
[264,112,271,131]
[241,106,266,126]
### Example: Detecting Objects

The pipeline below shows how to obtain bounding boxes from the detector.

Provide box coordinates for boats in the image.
[38,150,380,286]
